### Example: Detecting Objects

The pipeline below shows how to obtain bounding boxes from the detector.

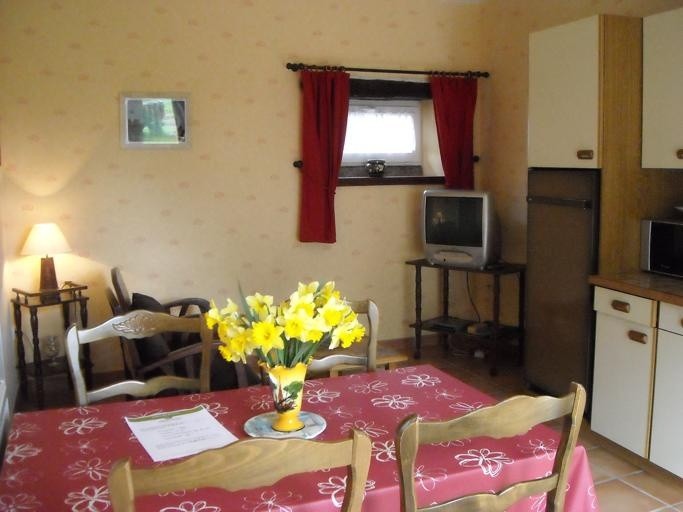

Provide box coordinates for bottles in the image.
[364,159,385,177]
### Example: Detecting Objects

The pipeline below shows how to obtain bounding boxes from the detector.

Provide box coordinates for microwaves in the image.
[638,217,682,277]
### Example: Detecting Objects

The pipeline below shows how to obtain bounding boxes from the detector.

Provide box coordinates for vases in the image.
[258,356,313,433]
[365,160,385,178]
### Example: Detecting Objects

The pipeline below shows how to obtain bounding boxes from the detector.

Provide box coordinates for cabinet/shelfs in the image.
[641,7,683,169]
[528,12,643,170]
[587,278,683,494]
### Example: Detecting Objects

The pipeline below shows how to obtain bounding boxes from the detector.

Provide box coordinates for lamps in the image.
[19,223,72,303]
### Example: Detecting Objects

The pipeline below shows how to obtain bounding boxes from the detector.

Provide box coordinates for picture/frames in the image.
[122,97,188,147]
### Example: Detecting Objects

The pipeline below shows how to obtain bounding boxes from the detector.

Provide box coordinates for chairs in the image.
[394,380,587,512]
[234,298,380,389]
[104,267,259,400]
[106,428,372,512]
[65,309,213,407]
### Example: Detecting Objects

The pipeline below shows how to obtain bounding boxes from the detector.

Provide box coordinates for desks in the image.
[0,363,600,512]
[405,257,527,376]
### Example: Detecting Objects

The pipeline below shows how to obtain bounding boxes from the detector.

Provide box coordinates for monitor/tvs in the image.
[421,189,501,272]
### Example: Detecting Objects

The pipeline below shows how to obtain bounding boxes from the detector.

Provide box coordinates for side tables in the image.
[10,281,94,409]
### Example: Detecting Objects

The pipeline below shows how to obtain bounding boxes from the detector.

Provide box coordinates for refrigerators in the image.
[519,167,598,415]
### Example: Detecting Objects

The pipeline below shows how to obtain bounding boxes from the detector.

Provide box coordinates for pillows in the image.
[129,293,176,377]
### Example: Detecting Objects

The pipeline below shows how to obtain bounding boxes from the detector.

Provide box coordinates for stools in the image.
[314,340,409,378]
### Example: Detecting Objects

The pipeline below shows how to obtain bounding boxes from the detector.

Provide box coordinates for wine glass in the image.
[43,334,63,368]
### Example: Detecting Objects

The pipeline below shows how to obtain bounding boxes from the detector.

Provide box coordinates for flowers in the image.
[202,281,366,365]
